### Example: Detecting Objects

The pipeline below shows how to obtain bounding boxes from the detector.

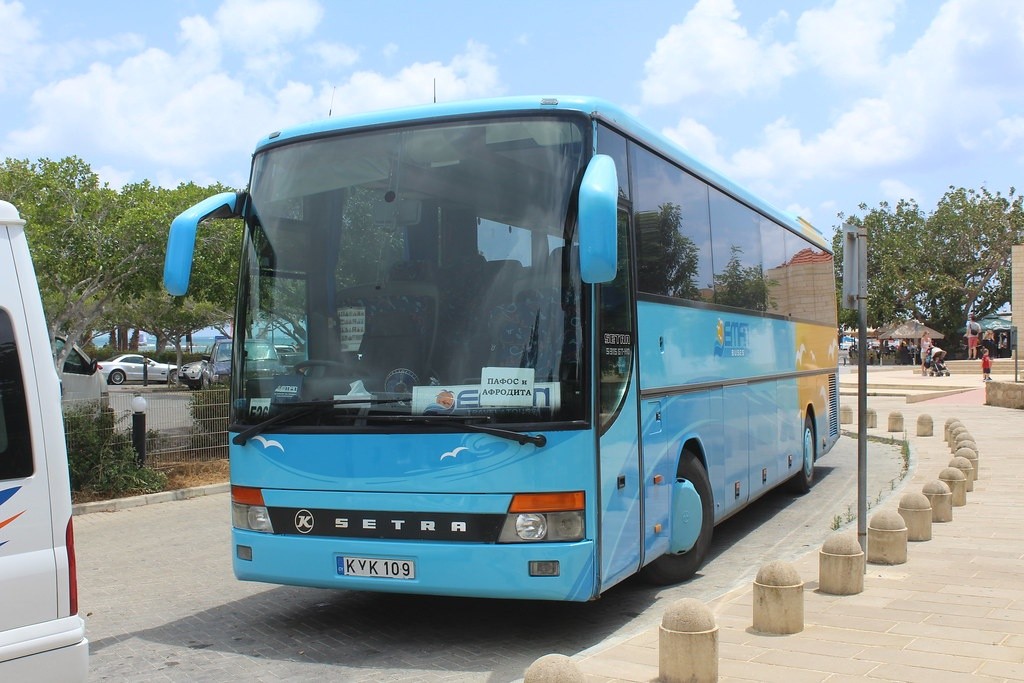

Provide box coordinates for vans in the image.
[0,199,89,682]
[49,329,115,448]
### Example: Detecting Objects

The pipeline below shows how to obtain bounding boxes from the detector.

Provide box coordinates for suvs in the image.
[201,338,289,392]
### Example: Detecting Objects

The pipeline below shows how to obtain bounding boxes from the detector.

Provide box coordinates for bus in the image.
[162,93,841,603]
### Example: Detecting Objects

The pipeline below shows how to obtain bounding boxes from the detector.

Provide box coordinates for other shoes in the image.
[983,378,987,382]
[974,358,976,360]
[968,357,971,360]
[987,377,992,380]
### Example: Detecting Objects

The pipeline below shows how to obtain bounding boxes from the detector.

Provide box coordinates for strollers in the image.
[922,345,951,377]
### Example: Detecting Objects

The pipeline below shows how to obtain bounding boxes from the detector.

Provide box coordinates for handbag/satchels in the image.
[924,355,931,368]
[971,330,978,335]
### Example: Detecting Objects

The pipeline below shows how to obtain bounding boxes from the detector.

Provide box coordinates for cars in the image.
[96,353,177,386]
[179,360,208,390]
[255,345,299,368]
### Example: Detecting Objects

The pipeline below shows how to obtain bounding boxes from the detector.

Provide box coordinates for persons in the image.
[935,356,947,368]
[983,330,995,360]
[980,349,992,380]
[921,331,934,376]
[966,316,981,360]
[995,332,1008,358]
